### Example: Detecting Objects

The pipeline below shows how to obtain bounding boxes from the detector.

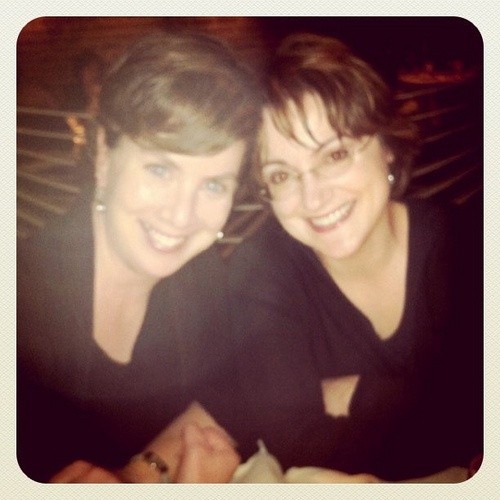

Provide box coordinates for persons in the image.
[49,35,484,484]
[17,34,263,485]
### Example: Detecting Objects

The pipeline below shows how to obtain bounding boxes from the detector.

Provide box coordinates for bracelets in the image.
[129,451,170,484]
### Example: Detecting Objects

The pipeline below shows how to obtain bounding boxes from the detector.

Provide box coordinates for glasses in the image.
[254,130,372,205]
